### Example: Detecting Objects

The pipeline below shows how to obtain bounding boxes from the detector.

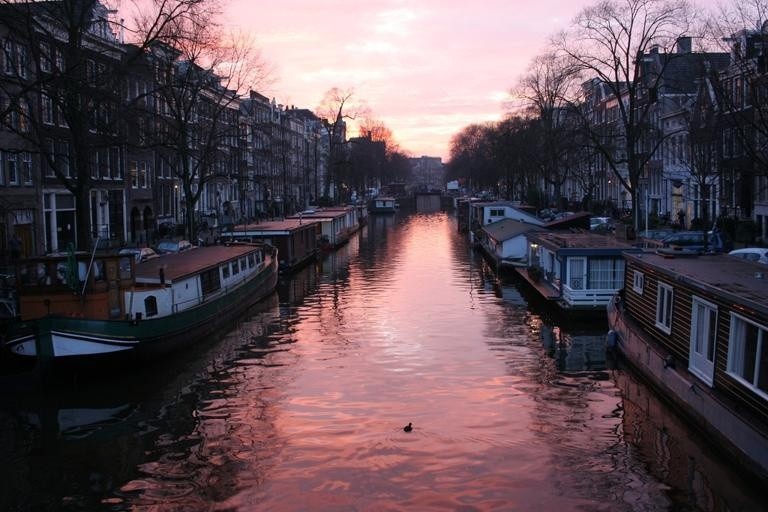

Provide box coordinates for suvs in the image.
[589,217,617,233]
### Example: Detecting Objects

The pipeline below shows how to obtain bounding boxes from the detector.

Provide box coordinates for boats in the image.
[0,236,279,395]
[605,245,768,490]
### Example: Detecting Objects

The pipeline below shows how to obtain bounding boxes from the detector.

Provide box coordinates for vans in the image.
[4,252,103,292]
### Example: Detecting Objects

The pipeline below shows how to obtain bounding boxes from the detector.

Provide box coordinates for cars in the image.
[118,240,199,272]
[639,229,768,265]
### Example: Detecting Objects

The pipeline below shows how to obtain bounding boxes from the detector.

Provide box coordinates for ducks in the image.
[404,421,413,432]
